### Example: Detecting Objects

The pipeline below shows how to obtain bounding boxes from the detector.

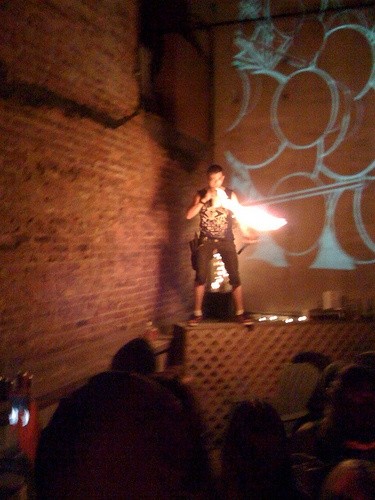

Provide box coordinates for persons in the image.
[0,334,375,500]
[185,164,261,328]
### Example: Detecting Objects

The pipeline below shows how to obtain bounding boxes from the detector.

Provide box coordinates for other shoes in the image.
[188,315,203,327]
[236,311,254,325]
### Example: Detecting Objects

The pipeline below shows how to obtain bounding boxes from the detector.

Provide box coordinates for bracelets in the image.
[199,200,206,205]
